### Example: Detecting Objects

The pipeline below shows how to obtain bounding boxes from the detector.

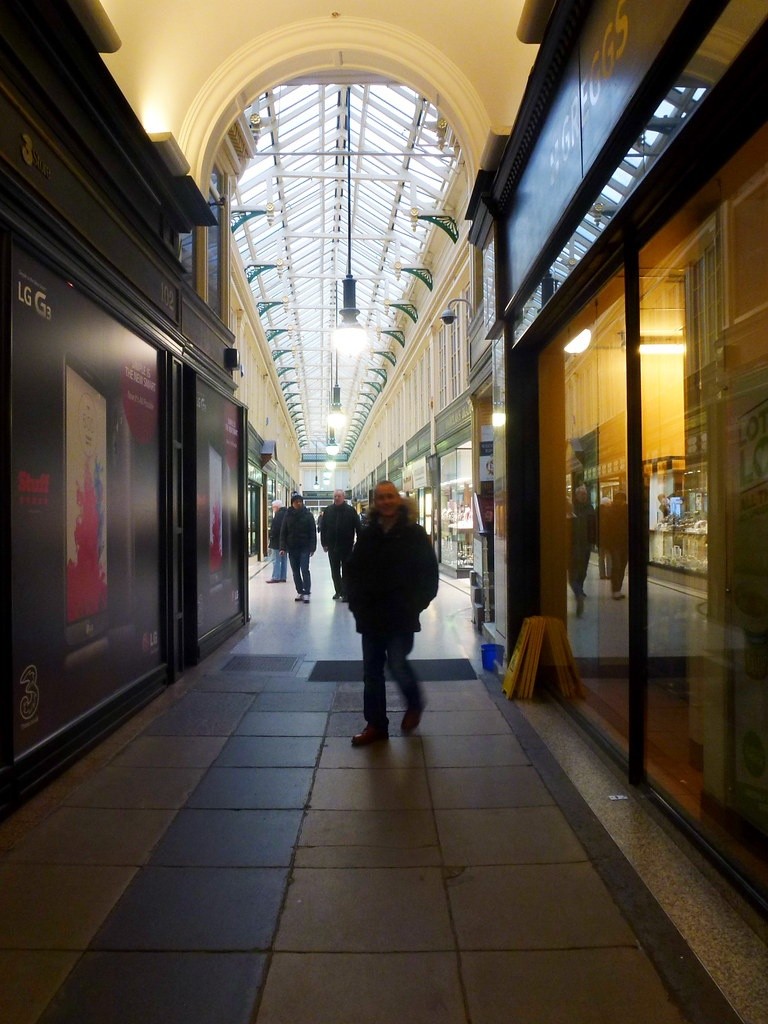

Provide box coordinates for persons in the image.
[605,493,629,600]
[658,494,677,518]
[277,495,318,603]
[593,496,613,579]
[265,500,289,583]
[317,511,325,533]
[358,507,369,525]
[321,488,362,604]
[565,486,597,616]
[346,480,440,745]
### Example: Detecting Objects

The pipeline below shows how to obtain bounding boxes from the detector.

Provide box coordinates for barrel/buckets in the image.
[480,644,505,670]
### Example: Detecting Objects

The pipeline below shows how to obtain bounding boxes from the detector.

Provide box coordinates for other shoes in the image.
[576,597,584,616]
[333,592,340,599]
[279,579,286,583]
[341,596,348,602]
[351,725,389,744]
[619,592,625,598]
[400,709,422,730]
[612,593,620,600]
[265,578,280,583]
[302,594,310,602]
[295,593,302,601]
[600,576,609,580]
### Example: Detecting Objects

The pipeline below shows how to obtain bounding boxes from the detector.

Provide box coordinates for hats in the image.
[291,490,303,505]
[615,489,626,500]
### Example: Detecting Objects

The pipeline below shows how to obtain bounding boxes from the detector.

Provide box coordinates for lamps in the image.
[337,86,361,329]
[322,281,341,482]
[440,298,472,325]
